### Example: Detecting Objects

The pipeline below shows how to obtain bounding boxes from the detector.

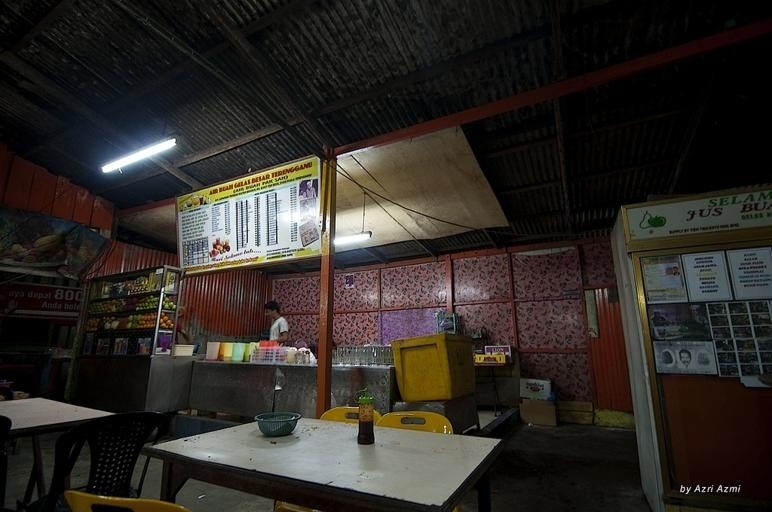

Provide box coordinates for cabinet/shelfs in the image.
[70,265,184,356]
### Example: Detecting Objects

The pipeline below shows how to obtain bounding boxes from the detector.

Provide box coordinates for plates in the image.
[1,256,66,268]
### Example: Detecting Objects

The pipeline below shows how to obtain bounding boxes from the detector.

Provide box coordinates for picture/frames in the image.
[638,244,772,306]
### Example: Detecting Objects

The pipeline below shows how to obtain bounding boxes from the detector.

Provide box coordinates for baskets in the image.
[253,409,302,438]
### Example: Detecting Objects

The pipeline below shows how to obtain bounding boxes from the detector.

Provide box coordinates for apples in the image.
[86,295,176,313]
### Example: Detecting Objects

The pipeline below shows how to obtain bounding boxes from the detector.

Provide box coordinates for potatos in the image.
[137,312,174,329]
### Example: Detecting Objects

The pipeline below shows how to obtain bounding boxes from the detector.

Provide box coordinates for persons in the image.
[678,349,692,368]
[263,300,289,345]
[302,180,317,197]
[671,265,680,276]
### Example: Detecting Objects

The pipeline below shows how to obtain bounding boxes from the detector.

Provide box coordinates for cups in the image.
[332,346,394,365]
[58,226,113,281]
[285,349,310,364]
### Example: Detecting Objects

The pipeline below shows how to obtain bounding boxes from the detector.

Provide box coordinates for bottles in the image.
[351,387,375,445]
[82,332,94,354]
[651,309,667,339]
[687,304,708,340]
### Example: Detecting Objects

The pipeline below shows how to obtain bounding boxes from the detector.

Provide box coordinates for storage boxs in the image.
[390,333,477,402]
[519,376,559,426]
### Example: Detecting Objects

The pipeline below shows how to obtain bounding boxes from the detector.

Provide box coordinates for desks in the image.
[140,416,505,511]
[0,396,118,512]
